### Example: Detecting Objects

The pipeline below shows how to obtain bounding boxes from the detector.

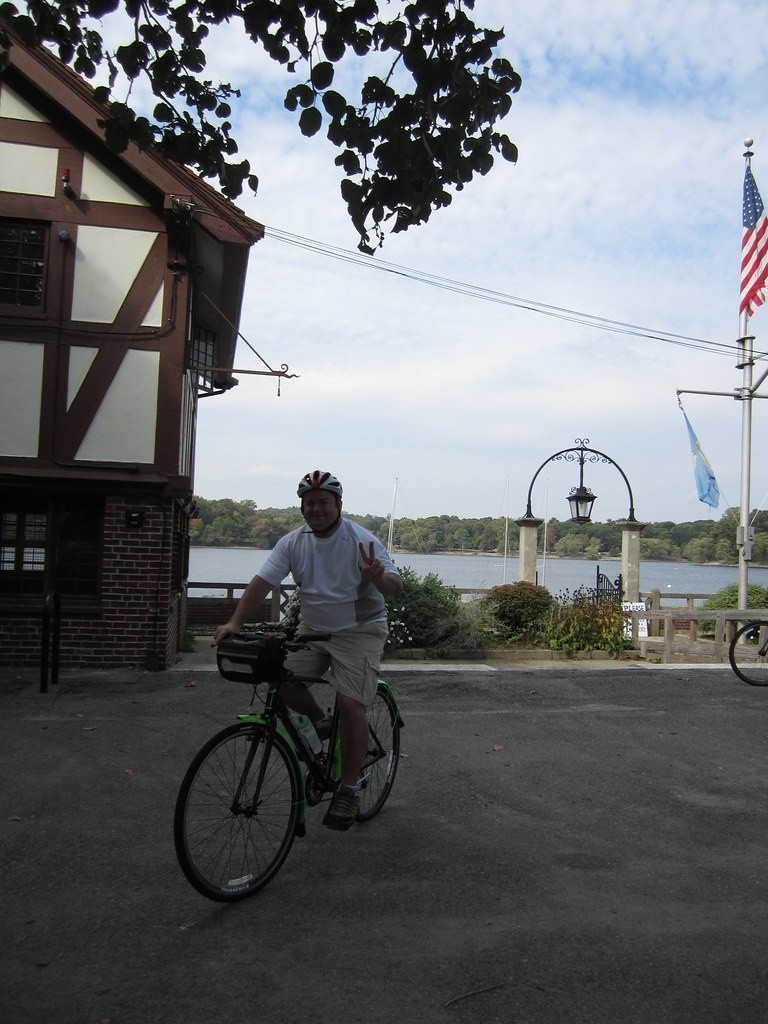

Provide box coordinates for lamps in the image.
[565,460,597,525]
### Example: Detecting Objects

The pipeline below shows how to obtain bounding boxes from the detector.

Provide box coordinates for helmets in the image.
[296,470,342,498]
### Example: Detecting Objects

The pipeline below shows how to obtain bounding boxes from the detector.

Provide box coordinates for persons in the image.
[213,470,404,832]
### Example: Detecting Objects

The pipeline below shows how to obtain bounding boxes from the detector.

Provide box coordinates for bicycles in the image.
[728,615,768,685]
[173,629,406,903]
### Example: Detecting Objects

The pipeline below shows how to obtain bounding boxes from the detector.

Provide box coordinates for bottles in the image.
[294,715,323,755]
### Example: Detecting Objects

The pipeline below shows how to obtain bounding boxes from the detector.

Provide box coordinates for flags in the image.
[739,166,768,322]
[678,397,721,509]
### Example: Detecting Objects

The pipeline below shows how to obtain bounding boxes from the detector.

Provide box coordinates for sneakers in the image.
[312,708,335,761]
[323,782,361,832]
